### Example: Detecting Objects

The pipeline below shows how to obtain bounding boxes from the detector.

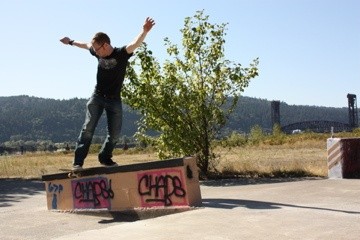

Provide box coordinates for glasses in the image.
[93,45,103,52]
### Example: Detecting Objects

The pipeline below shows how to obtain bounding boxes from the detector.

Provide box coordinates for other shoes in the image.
[72,164,82,169]
[99,159,118,167]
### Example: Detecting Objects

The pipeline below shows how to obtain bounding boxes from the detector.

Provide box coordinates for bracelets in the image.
[68,40,74,45]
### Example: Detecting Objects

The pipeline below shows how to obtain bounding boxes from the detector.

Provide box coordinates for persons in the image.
[59,16,156,172]
